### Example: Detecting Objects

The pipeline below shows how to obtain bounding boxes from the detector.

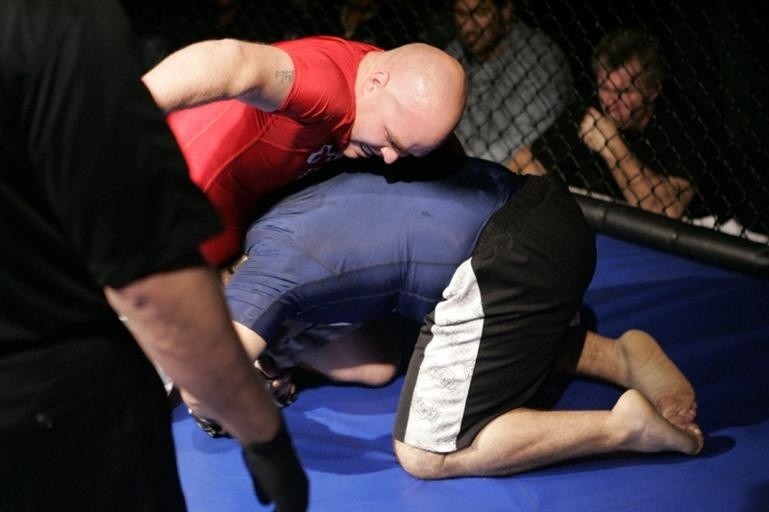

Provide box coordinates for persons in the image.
[142,2,767,243]
[179,149,704,478]
[141,32,470,442]
[0,0,310,512]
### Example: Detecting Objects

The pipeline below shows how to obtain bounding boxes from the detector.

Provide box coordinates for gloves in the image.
[242,419,309,512]
